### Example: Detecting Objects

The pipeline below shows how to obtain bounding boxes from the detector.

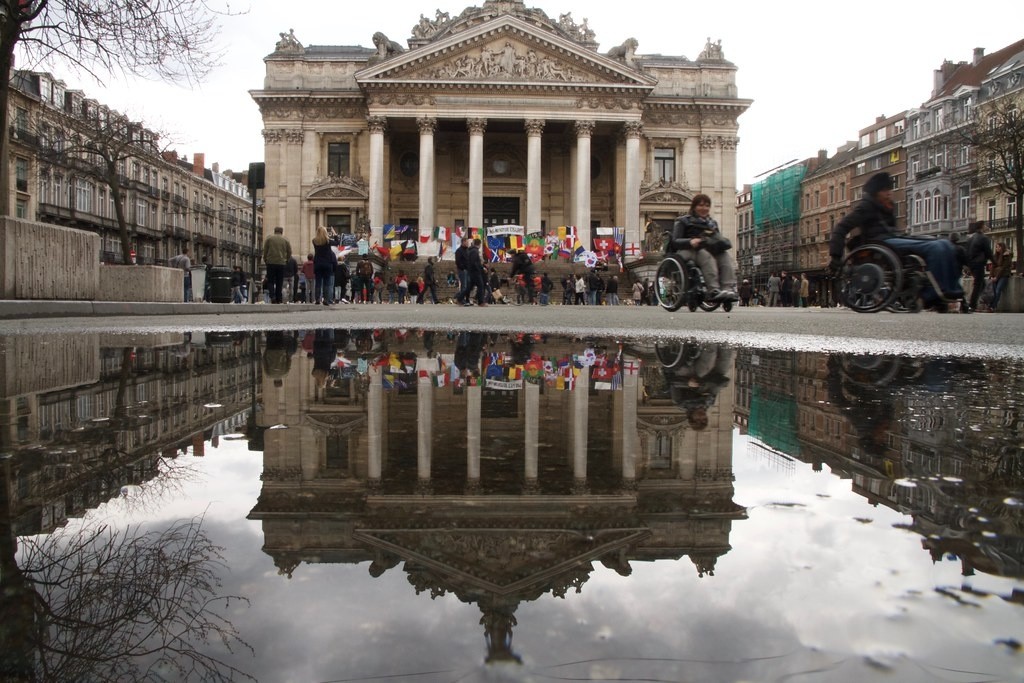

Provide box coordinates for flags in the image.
[328,223,641,273]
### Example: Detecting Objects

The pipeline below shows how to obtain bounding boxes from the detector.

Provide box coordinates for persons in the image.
[194,250,667,306]
[946,232,969,313]
[828,171,966,301]
[964,220,998,312]
[456,238,491,307]
[168,248,193,302]
[455,237,470,307]
[171,332,940,457]
[737,271,809,309]
[986,242,1014,313]
[262,226,292,304]
[312,226,340,307]
[671,194,737,302]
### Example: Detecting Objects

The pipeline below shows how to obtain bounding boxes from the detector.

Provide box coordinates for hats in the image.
[863,172,894,193]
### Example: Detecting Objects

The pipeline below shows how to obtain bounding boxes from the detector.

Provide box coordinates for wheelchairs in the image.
[835,353,931,392]
[653,230,739,313]
[653,341,733,392]
[835,231,964,314]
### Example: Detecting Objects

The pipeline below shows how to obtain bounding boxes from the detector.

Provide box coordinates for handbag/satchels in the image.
[399,279,408,289]
[699,229,731,253]
[375,281,384,289]
[492,289,502,300]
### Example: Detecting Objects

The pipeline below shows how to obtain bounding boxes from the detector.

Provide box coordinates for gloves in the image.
[828,255,842,274]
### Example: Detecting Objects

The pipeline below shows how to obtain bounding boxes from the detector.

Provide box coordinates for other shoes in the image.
[323,302,329,306]
[435,301,442,304]
[416,299,423,304]
[230,300,234,304]
[988,307,994,312]
[943,289,965,299]
[725,290,735,299]
[479,302,489,307]
[709,288,727,300]
[466,303,470,306]
[241,299,246,304]
[342,298,347,303]
[315,302,320,305]
